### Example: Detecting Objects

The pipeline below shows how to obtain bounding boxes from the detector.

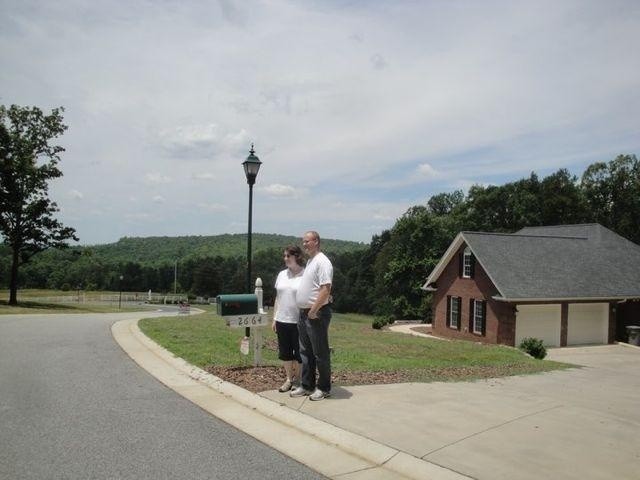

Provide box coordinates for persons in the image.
[271,245,307,393]
[289,231,336,400]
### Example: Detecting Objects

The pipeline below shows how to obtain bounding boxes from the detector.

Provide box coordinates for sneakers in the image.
[279,380,331,401]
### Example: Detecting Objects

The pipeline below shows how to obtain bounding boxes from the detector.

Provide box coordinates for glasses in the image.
[283,254,294,258]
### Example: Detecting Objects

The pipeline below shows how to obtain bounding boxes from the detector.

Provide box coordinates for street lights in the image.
[241,144,261,367]
[119,276,123,309]
